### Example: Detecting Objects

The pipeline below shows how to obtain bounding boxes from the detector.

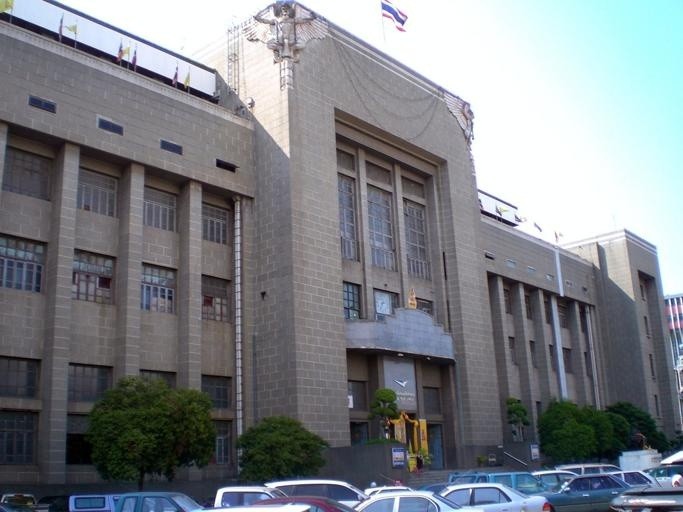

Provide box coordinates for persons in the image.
[394,479,402,485]
[642,436,651,450]
[415,454,423,479]
[670,473,683,487]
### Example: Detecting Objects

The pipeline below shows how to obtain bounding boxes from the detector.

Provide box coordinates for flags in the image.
[381,0,408,32]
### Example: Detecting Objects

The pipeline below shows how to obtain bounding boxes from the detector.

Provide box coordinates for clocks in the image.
[373,289,393,317]
